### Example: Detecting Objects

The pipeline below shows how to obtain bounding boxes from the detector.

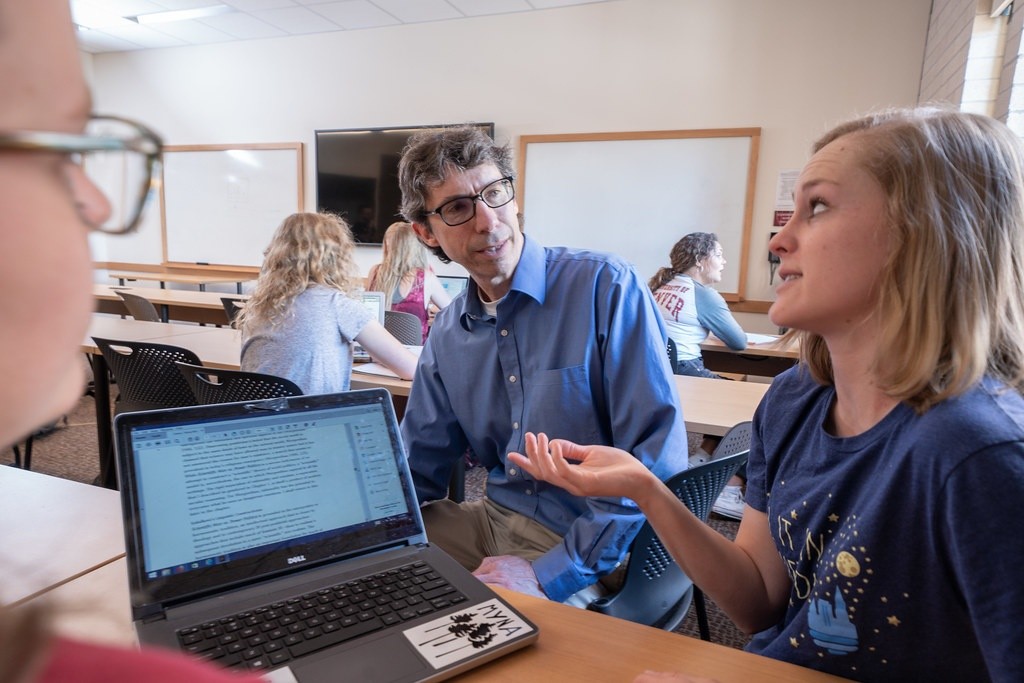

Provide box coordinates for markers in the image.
[196,262,208,265]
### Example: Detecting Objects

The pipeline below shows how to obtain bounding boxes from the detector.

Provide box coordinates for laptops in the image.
[350,291,385,362]
[436,276,469,310]
[113,387,539,683]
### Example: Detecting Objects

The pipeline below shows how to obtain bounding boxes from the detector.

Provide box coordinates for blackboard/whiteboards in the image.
[158,142,305,274]
[516,125,763,303]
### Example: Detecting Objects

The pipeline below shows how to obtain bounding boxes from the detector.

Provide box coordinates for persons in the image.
[509,107,1024,683]
[648,232,747,522]
[236,213,418,397]
[0,0,275,683]
[399,127,688,613]
[366,222,452,346]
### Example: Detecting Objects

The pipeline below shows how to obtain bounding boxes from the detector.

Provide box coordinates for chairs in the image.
[384,310,423,346]
[113,290,161,322]
[588,420,752,637]
[666,338,678,371]
[174,360,304,407]
[220,297,248,329]
[90,336,211,419]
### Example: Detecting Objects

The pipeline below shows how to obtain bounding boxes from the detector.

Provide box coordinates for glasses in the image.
[3,110,167,239]
[424,173,515,227]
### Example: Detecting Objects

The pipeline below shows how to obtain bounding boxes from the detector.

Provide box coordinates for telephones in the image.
[768,232,782,264]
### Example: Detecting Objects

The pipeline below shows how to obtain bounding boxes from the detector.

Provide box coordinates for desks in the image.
[74,270,414,480]
[0,463,125,605]
[699,332,800,359]
[673,373,773,436]
[18,555,850,683]
